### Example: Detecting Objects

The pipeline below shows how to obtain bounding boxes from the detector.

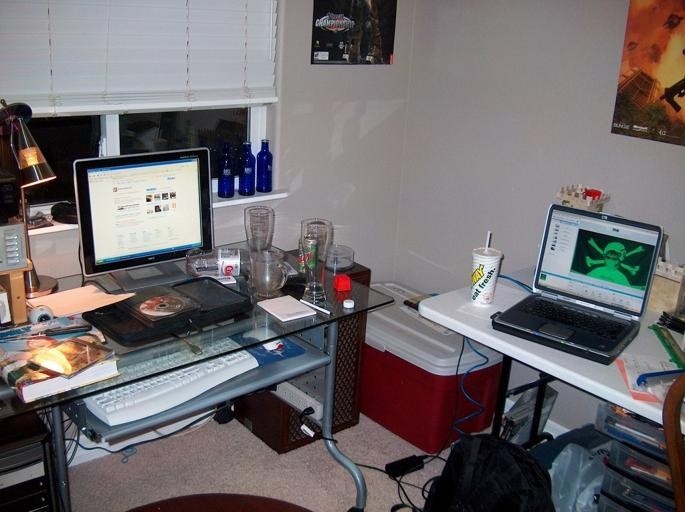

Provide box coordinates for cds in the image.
[139,295,186,318]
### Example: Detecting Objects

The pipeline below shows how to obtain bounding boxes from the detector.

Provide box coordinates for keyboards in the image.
[75,336,260,429]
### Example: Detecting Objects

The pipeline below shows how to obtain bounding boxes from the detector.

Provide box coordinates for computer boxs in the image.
[0,411,59,512]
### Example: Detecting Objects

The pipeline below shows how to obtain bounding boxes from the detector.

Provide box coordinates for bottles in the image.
[256,141,273,193]
[184,248,250,278]
[344,1,383,64]
[216,143,234,199]
[238,139,256,196]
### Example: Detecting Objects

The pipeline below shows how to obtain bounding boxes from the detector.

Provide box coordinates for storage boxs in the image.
[357,280,504,455]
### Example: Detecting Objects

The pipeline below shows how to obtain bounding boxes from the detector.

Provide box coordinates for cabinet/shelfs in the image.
[592,401,685,512]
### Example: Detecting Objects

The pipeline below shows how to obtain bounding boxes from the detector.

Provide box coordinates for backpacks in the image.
[423,433,555,512]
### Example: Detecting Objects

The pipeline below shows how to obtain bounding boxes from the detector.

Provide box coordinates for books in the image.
[0,336,120,404]
[403,292,440,311]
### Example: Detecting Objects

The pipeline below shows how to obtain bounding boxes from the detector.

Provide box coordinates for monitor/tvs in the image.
[72,147,218,292]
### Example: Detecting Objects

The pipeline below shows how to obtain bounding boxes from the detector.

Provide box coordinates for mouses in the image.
[242,327,284,352]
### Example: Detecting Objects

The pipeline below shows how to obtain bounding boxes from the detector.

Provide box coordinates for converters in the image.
[385,454,425,479]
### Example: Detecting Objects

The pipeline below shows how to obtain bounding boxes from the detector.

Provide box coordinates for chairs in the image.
[662,373,685,512]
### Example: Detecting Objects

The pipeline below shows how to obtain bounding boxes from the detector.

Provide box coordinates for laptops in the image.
[492,202,665,367]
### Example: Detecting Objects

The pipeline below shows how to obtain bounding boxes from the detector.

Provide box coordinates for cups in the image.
[300,217,333,300]
[243,202,274,254]
[250,251,289,300]
[471,246,502,306]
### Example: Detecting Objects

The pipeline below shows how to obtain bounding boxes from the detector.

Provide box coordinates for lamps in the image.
[1,97,61,299]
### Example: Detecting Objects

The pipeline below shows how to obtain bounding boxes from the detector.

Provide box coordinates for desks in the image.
[1,236,396,512]
[417,267,685,451]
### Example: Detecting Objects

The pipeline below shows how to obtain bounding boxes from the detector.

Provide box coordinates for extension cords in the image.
[269,382,322,421]
